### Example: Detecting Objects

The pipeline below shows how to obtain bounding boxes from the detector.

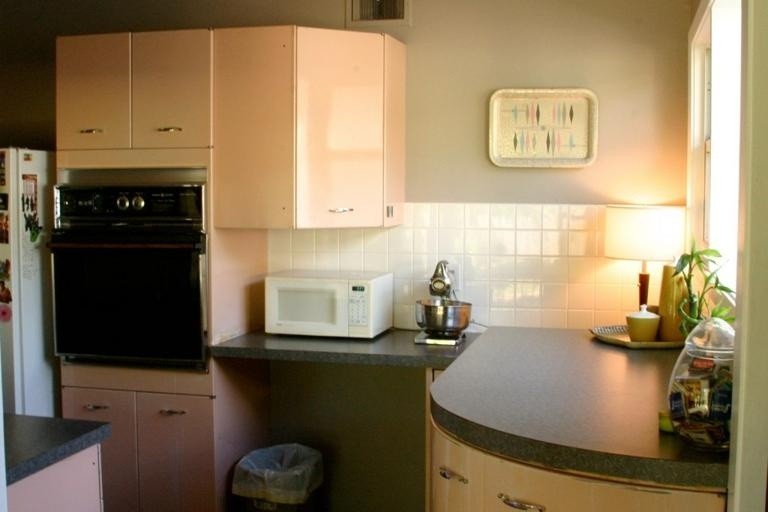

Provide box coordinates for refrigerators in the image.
[0,147,61,421]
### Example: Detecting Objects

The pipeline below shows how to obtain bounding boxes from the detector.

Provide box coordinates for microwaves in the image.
[265,269,394,340]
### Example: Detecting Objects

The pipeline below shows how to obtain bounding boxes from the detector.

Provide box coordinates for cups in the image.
[626,304,661,342]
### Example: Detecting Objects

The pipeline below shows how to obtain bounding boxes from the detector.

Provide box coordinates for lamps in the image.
[602,203,681,312]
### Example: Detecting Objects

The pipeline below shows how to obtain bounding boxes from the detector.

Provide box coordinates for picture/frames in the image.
[345,0,412,28]
[489,88,599,168]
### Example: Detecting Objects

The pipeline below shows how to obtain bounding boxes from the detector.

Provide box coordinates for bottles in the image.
[661,257,692,341]
[667,316,737,450]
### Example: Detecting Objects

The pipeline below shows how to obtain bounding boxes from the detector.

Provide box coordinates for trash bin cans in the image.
[232,444,323,512]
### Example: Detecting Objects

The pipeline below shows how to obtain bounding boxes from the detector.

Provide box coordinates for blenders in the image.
[414,261,472,345]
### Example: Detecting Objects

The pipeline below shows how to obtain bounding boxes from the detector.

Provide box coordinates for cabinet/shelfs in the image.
[55,28,214,170]
[214,25,407,231]
[60,360,265,512]
[429,414,726,512]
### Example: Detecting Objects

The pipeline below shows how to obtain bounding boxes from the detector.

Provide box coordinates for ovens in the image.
[50,183,209,370]
